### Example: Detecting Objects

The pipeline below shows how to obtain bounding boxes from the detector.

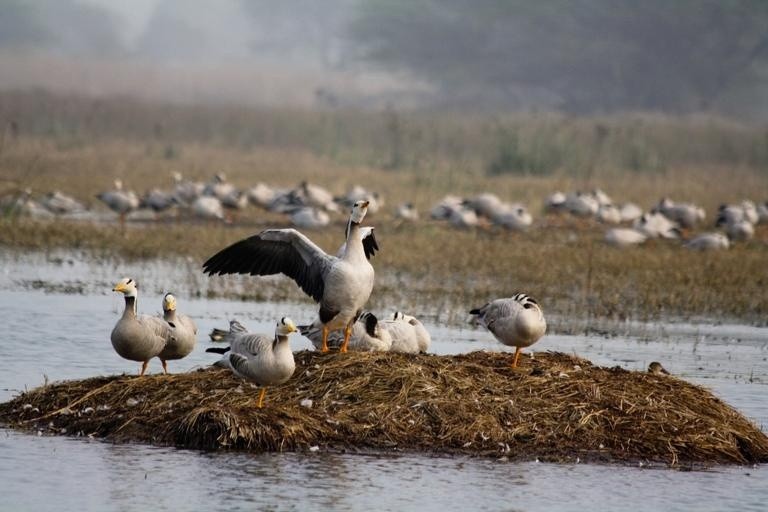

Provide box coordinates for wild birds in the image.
[92,169,768,253]
[41,190,90,219]
[0,186,37,212]
[152,292,198,375]
[110,277,176,377]
[468,292,547,368]
[223,315,299,407]
[377,310,431,355]
[295,307,393,353]
[201,200,379,353]
